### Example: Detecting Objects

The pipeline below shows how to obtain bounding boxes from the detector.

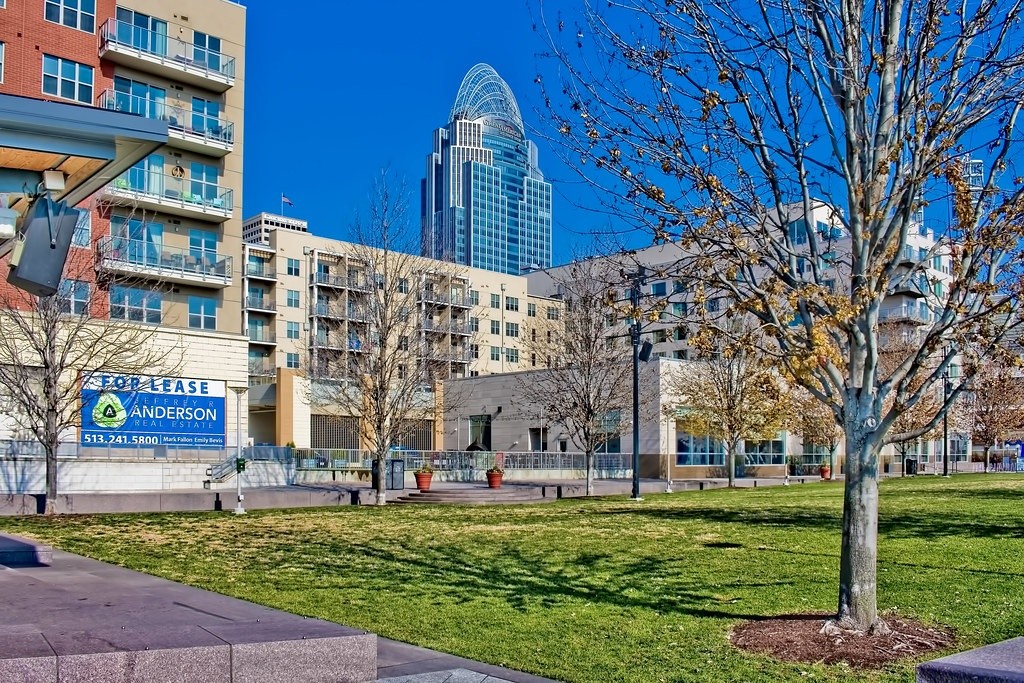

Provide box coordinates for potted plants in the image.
[413,463,435,490]
[819,460,832,480]
[487,466,505,488]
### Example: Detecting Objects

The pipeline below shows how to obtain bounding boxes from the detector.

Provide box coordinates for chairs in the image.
[114,176,224,209]
[160,250,216,276]
[168,116,184,129]
[211,125,224,137]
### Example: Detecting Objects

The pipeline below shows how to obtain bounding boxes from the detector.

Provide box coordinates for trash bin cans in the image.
[372,458,405,490]
[905,458,917,476]
